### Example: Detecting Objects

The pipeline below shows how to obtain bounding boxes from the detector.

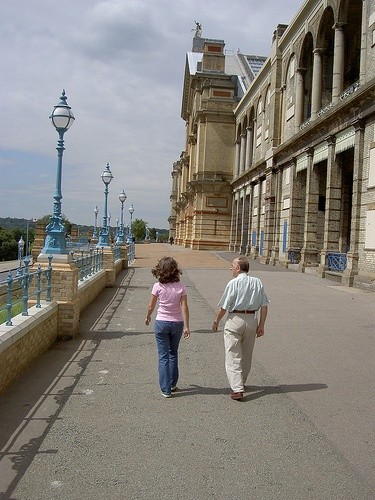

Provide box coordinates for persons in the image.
[170,236,174,246]
[144,258,190,399]
[212,257,271,400]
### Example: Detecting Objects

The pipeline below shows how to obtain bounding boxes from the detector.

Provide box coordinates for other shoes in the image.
[162,391,172,398]
[171,386,177,391]
[231,392,243,400]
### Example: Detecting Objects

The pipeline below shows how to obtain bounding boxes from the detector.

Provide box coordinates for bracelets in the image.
[214,320,219,324]
[145,315,151,320]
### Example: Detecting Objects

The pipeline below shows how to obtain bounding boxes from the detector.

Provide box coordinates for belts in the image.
[230,310,255,314]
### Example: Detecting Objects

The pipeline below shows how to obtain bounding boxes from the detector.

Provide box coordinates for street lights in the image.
[108,215,111,233]
[97,163,114,246]
[116,219,119,229]
[128,202,135,242]
[116,189,127,246]
[39,90,75,254]
[16,236,25,277]
[92,206,99,237]
